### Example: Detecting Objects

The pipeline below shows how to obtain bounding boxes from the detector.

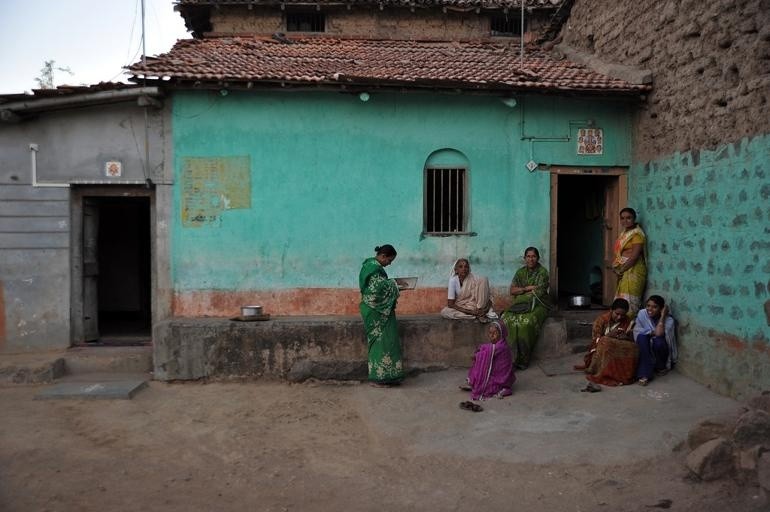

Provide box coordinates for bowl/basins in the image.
[241,306,262,317]
[570,295,591,308]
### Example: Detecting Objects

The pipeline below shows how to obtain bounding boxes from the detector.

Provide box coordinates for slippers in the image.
[580,382,601,393]
[459,401,483,412]
[639,377,649,386]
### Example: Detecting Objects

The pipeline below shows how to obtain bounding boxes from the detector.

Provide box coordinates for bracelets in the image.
[658,321,664,325]
[526,286,529,292]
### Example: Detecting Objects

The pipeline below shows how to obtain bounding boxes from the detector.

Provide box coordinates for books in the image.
[388,276,418,290]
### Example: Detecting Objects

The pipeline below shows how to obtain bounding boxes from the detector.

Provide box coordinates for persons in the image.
[610,207,647,308]
[442,257,493,324]
[572,297,633,381]
[497,246,553,371]
[578,129,602,154]
[634,295,681,385]
[456,318,517,397]
[358,244,408,386]
